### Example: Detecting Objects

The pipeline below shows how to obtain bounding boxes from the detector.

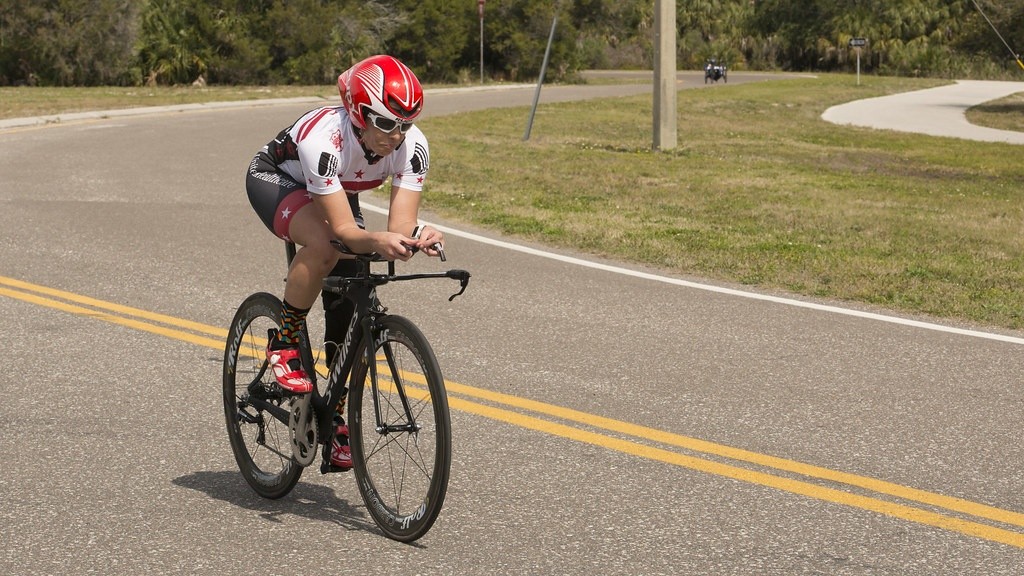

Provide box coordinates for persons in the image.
[246,54,445,468]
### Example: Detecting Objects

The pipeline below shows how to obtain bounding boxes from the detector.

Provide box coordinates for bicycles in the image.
[222,239,471,544]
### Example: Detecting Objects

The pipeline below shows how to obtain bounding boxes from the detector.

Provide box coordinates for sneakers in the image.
[265,328,313,394]
[322,416,353,468]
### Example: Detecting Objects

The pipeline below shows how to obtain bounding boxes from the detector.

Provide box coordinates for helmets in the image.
[338,54,424,130]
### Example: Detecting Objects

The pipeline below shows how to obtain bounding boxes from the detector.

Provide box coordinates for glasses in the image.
[366,112,412,135]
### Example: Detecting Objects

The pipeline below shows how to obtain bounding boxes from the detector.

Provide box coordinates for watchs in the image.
[411,224,427,238]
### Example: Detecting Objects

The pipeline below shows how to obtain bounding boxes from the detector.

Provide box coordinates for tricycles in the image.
[703,59,727,84]
